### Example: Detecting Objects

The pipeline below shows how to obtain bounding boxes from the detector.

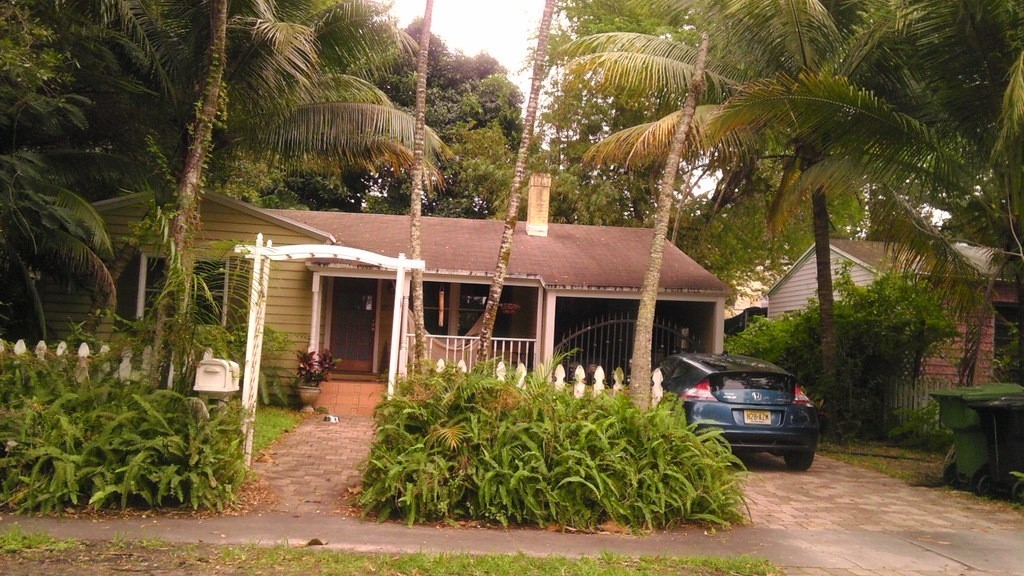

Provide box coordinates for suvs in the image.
[650,355,817,471]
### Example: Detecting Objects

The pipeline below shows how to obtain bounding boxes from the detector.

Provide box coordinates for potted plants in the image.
[295,349,342,412]
[499,303,521,315]
